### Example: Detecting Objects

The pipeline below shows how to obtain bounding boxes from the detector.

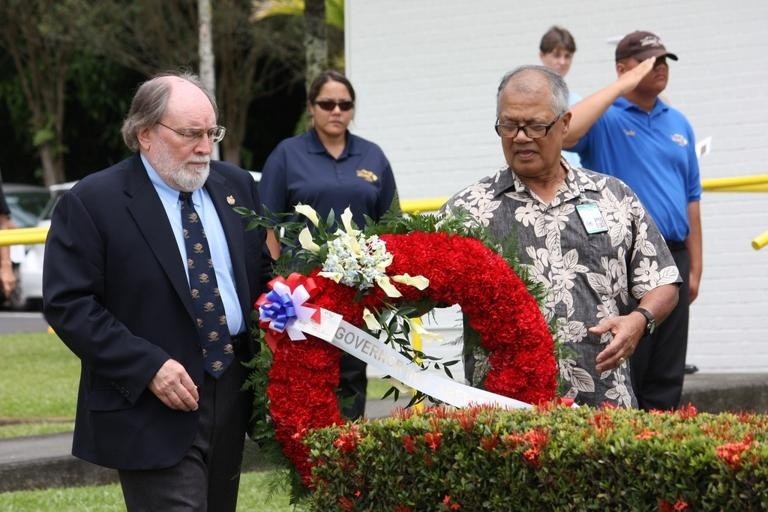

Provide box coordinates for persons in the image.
[41,69,281,511]
[0,182,18,302]
[256,70,407,424]
[402,67,685,412]
[538,23,585,168]
[561,29,704,408]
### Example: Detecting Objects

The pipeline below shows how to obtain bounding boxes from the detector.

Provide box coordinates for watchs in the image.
[630,307,658,341]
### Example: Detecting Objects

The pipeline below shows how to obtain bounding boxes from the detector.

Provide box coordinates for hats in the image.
[615,30,677,62]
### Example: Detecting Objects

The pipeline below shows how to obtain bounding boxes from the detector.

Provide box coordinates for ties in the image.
[178,191,234,379]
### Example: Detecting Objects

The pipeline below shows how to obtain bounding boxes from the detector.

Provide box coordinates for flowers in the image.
[225,194,575,512]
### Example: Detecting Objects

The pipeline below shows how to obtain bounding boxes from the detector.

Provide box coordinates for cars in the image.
[0,184,48,253]
[6,181,87,308]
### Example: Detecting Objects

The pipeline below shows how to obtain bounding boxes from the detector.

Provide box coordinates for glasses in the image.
[311,101,353,110]
[155,121,226,143]
[494,112,563,139]
